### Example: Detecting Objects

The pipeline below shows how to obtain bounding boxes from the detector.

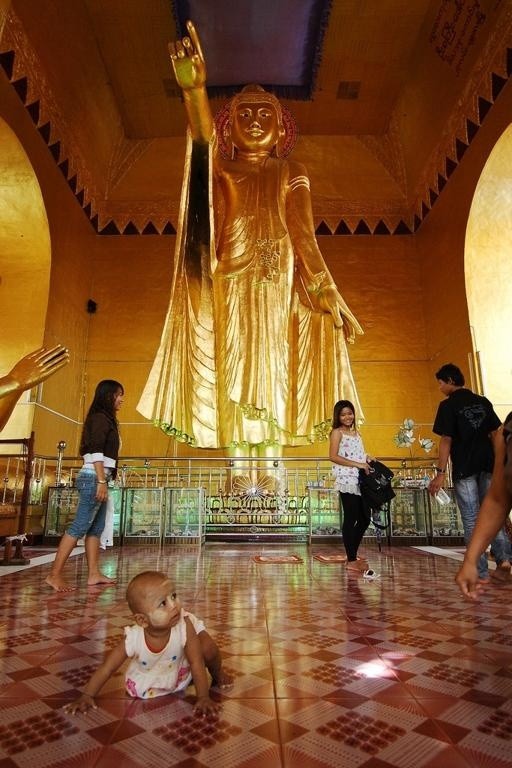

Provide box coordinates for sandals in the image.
[497,558,511,570]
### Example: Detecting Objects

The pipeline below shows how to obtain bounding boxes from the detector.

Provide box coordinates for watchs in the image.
[96,479,108,484]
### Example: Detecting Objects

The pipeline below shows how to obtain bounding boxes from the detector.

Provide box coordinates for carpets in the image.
[250,548,363,564]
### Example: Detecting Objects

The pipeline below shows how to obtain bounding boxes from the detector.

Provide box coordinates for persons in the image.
[455,411,512,600]
[45,379,125,593]
[330,401,378,573]
[0,342,70,432]
[133,21,367,512]
[62,571,234,719]
[427,362,511,583]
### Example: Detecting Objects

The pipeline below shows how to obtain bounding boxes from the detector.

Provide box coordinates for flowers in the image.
[392,419,434,475]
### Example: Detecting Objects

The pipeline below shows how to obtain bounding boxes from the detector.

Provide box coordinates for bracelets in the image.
[438,468,446,476]
[352,461,357,468]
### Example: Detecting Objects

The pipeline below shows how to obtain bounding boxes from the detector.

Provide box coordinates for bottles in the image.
[424,475,451,506]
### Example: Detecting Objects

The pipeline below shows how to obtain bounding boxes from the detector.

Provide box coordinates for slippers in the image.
[345,559,369,572]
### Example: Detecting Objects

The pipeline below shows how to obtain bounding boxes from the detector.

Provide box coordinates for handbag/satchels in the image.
[358,460,396,509]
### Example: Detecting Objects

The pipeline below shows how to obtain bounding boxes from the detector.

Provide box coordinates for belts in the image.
[81,462,114,477]
